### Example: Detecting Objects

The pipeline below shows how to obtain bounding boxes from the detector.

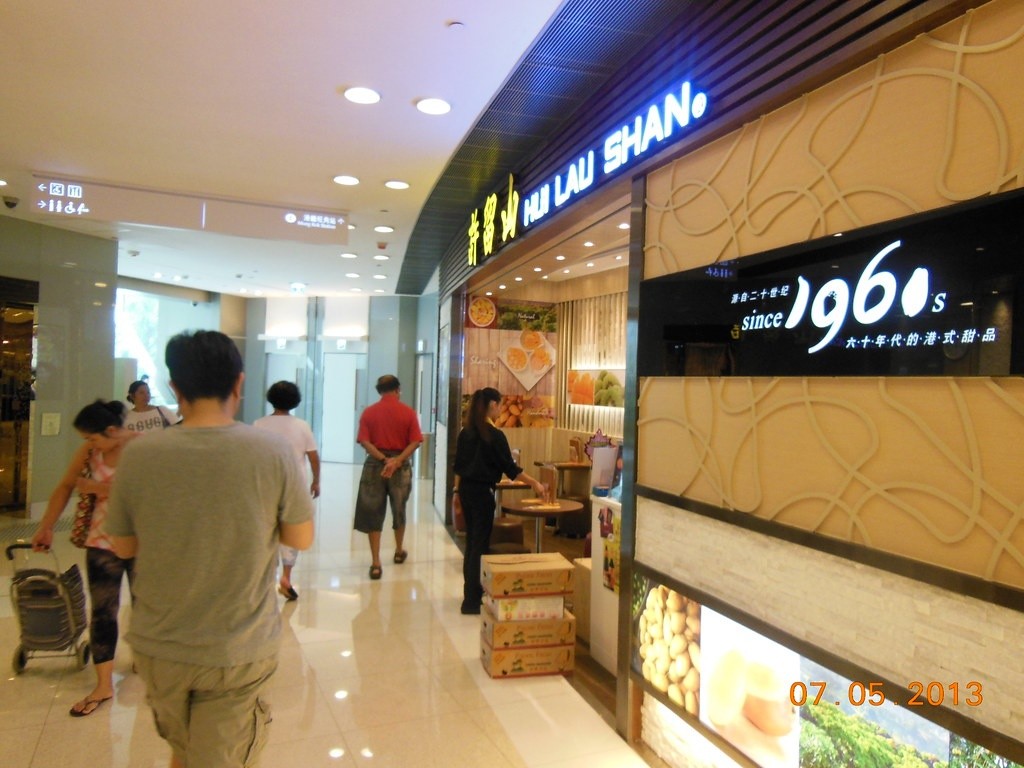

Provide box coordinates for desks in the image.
[500,496,584,554]
[494,482,530,527]
[533,459,591,532]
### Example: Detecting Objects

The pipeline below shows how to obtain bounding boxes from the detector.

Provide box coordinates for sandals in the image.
[395,549,408,563]
[370,565,380,578]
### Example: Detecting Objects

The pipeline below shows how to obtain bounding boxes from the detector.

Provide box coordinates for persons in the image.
[102,328,314,768]
[600,508,613,538]
[250,381,320,600]
[352,375,424,580]
[461,409,468,428]
[32,398,141,719]
[461,390,470,406]
[452,387,546,615]
[120,381,179,431]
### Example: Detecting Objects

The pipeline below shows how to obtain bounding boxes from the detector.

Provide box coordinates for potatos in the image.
[640,585,707,716]
[709,651,794,736]
[493,394,526,427]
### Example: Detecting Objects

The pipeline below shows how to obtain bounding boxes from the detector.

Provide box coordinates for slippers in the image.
[68,692,112,717]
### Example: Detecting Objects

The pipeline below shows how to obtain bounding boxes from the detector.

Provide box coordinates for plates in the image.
[496,330,557,392]
[468,296,496,327]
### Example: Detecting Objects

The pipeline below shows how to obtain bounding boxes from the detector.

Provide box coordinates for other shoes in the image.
[279,583,300,603]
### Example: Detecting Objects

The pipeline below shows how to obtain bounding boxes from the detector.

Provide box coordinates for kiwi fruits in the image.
[595,370,625,410]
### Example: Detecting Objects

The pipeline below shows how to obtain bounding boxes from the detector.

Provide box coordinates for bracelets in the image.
[453,487,458,492]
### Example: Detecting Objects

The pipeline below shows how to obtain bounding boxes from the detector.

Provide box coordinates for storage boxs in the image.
[479,551,576,597]
[478,632,576,679]
[480,600,576,649]
[484,589,565,622]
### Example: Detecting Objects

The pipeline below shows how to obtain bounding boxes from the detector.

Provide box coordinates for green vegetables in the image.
[498,303,557,332]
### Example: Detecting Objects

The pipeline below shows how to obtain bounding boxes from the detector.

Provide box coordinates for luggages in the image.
[6,545,90,674]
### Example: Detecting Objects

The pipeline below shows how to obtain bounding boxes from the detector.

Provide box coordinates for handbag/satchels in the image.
[70,444,94,545]
